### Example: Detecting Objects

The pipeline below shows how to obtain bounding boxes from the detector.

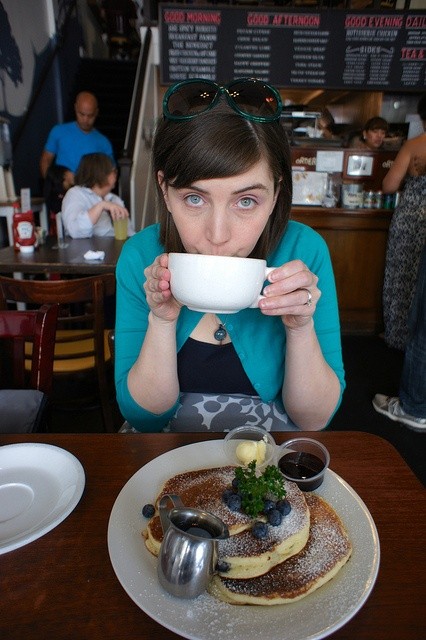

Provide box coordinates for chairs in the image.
[1,276,116,395]
[0,306,56,433]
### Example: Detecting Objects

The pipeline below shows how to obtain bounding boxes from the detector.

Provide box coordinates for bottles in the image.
[385,196,391,209]
[321,156,340,208]
[392,192,400,209]
[374,191,383,209]
[364,188,373,209]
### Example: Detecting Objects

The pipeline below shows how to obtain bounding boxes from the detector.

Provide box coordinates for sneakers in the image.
[373,393,426,432]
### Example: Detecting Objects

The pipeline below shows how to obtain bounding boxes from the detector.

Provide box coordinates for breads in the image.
[145,465,311,578]
[205,492,354,604]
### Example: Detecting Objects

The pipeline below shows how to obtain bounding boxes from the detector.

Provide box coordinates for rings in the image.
[304,288,313,306]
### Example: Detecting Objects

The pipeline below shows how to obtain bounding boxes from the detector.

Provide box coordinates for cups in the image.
[153,492,231,601]
[57,212,68,247]
[113,212,128,241]
[167,251,279,315]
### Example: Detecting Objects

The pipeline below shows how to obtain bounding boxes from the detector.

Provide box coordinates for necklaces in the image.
[213,315,230,343]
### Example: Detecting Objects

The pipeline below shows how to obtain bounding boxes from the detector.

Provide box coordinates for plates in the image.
[105,439,383,636]
[1,441,87,553]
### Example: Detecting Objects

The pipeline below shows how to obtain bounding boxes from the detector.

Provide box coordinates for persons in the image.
[381,96,425,347]
[351,115,386,151]
[39,92,115,182]
[372,246,426,431]
[62,152,130,238]
[113,76,348,433]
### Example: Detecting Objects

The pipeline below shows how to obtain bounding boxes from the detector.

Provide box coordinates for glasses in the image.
[108,169,120,174]
[163,79,283,123]
